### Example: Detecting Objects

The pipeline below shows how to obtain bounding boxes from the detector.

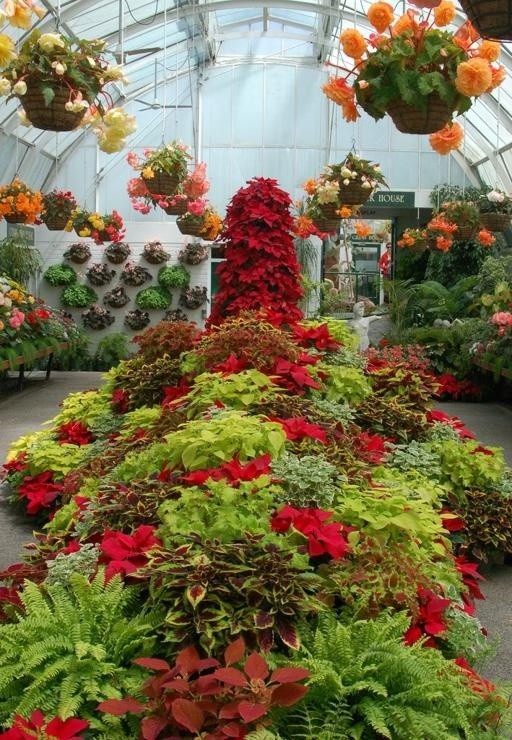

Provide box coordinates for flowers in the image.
[322,0,510,164]
[127,139,225,244]
[133,173,350,354]
[1,0,142,157]
[0,268,80,349]
[362,310,509,400]
[105,354,328,404]
[1,174,123,245]
[289,150,387,247]
[376,190,511,283]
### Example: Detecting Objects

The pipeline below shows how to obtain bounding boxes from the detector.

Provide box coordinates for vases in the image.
[12,76,85,134]
[380,82,453,138]
